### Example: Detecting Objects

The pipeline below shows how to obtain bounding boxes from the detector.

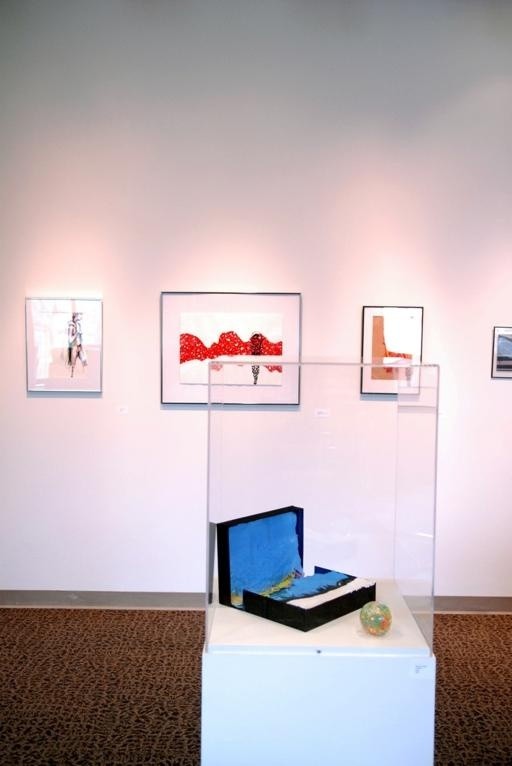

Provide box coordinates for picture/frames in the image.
[490,325,512,379]
[25,295,104,394]
[360,305,423,396]
[159,289,301,406]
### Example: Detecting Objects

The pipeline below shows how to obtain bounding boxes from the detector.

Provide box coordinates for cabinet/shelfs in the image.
[197,362,439,766]
